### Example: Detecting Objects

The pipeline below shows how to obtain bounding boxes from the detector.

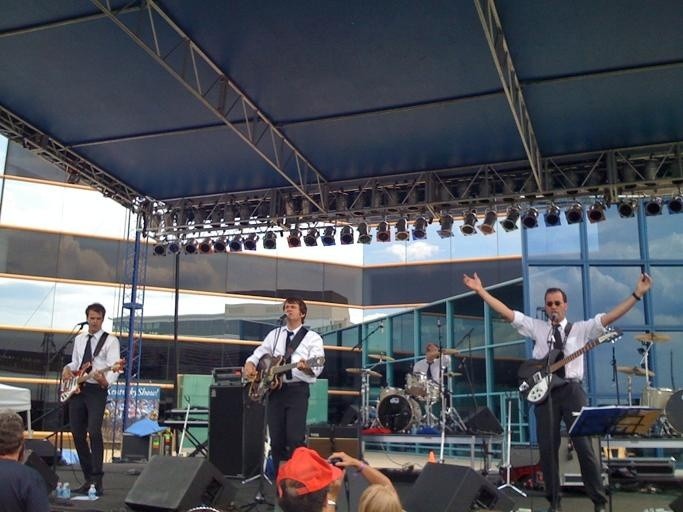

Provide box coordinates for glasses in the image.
[547,301,560,306]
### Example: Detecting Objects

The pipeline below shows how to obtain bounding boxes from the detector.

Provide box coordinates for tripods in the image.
[233,379,277,512]
[492,399,529,499]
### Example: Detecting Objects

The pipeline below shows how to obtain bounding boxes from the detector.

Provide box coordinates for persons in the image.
[240,297,326,487]
[0,408,49,512]
[61,302,121,495]
[412,343,452,413]
[275,443,345,512]
[461,271,654,512]
[322,448,404,512]
[102,400,158,425]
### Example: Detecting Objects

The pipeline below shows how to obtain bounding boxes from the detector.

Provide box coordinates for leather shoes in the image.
[71,482,103,495]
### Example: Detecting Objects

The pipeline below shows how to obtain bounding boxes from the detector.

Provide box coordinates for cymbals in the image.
[443,372,462,377]
[441,348,461,355]
[368,354,394,360]
[345,368,382,378]
[634,334,671,342]
[617,365,656,377]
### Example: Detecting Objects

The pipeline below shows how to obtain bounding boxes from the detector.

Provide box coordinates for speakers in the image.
[209,383,266,481]
[404,462,514,512]
[463,406,505,435]
[19,450,59,496]
[25,438,61,467]
[124,454,239,512]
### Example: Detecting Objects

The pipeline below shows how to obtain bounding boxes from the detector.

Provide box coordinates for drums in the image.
[639,386,682,437]
[663,389,683,436]
[376,394,422,434]
[404,371,427,398]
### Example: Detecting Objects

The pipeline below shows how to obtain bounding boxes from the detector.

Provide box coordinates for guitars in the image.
[239,354,325,403]
[516,328,622,401]
[57,357,127,404]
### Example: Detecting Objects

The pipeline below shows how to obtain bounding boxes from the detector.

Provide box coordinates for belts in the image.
[565,379,579,383]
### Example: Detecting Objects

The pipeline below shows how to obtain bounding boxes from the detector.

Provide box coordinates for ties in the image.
[553,325,565,378]
[81,334,92,374]
[286,331,293,379]
[427,363,431,377]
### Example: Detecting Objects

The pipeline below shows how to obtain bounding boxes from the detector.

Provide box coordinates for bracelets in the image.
[325,499,336,505]
[631,291,641,301]
[358,461,365,473]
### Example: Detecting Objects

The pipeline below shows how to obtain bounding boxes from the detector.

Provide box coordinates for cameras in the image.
[330,458,345,470]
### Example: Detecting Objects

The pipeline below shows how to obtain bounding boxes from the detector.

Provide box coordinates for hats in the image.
[276,447,342,497]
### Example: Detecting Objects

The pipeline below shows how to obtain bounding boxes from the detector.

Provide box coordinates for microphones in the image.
[551,311,558,321]
[277,312,289,322]
[455,327,475,347]
[437,316,441,327]
[380,320,384,333]
[76,320,90,326]
[457,357,467,369]
